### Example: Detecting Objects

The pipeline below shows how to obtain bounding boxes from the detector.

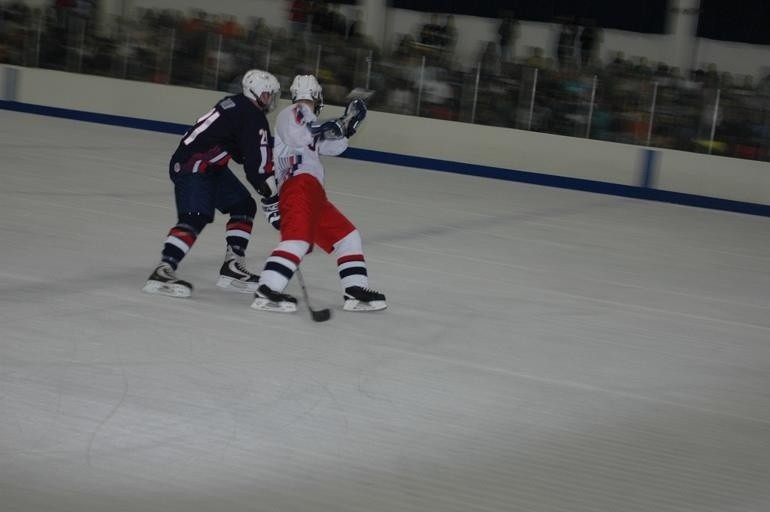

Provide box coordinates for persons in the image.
[1,0,305,99]
[251,72,386,315]
[292,0,770,163]
[142,68,281,297]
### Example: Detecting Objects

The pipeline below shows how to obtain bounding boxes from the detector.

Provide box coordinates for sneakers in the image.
[343,286,385,301]
[255,284,297,304]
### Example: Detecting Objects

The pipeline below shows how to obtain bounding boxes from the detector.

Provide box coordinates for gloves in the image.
[309,118,343,141]
[262,195,281,230]
[340,98,367,137]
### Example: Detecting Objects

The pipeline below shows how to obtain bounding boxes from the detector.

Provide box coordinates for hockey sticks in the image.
[292,262,330,323]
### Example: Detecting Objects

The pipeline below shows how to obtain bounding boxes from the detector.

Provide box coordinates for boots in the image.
[220,241,261,282]
[148,258,193,291]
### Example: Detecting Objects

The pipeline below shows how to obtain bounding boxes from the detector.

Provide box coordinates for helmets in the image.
[289,74,324,114]
[243,69,281,112]
